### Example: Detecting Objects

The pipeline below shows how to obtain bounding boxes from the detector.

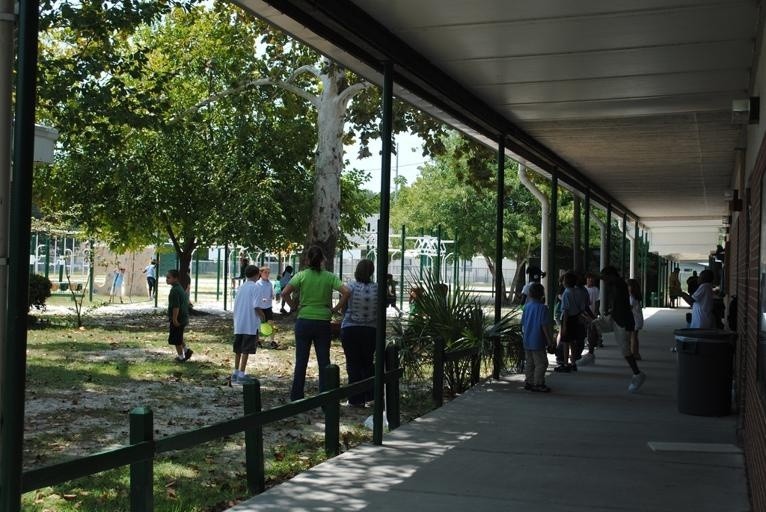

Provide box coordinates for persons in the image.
[108,267,126,305]
[408,288,426,318]
[273,273,281,304]
[138,257,157,302]
[165,269,193,364]
[385,273,403,317]
[231,252,248,289]
[280,266,292,313]
[256,266,278,348]
[185,268,192,306]
[340,258,378,409]
[230,265,265,384]
[520,265,727,394]
[281,245,352,414]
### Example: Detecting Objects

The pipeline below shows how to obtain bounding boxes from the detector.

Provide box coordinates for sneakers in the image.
[628,371,647,393]
[523,382,550,392]
[575,352,596,366]
[554,362,577,372]
[172,357,184,363]
[340,399,375,409]
[231,374,257,382]
[184,348,193,360]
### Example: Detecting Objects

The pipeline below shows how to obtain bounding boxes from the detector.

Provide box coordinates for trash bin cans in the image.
[673,328,739,417]
